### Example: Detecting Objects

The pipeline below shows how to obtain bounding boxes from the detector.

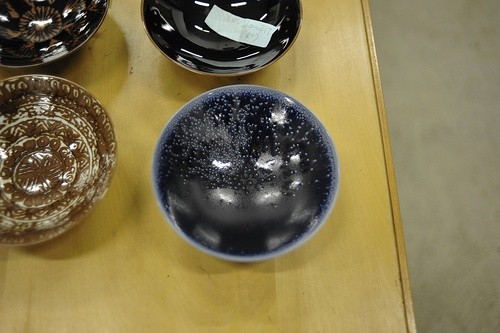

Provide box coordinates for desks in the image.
[0,0,415,333]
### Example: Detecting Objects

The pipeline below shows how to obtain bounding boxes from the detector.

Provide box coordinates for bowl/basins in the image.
[0,0,110,68]
[139,0,303,77]
[151,85,340,263]
[0,75,117,248]
